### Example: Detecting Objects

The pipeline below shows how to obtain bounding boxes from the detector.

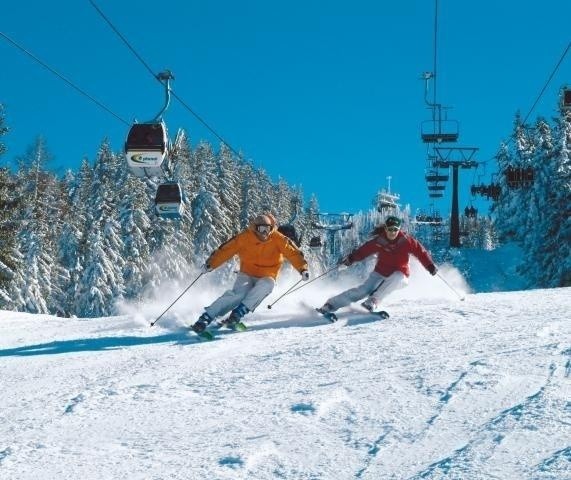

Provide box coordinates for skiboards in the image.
[316,308,389,323]
[191,323,247,340]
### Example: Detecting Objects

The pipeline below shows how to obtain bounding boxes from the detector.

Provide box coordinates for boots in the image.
[321,303,333,313]
[361,297,377,311]
[228,303,251,322]
[195,314,212,328]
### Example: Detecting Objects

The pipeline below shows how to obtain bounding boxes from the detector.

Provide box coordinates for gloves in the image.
[430,264,438,276]
[302,271,309,281]
[343,256,352,266]
[201,264,211,274]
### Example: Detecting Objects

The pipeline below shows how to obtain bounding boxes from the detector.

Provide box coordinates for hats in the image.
[386,216,401,229]
[256,215,271,224]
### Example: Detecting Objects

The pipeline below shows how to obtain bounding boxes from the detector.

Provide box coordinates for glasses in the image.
[256,225,271,234]
[386,227,400,231]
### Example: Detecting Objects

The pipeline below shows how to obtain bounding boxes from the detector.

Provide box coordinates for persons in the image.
[319,216,440,314]
[192,212,310,333]
